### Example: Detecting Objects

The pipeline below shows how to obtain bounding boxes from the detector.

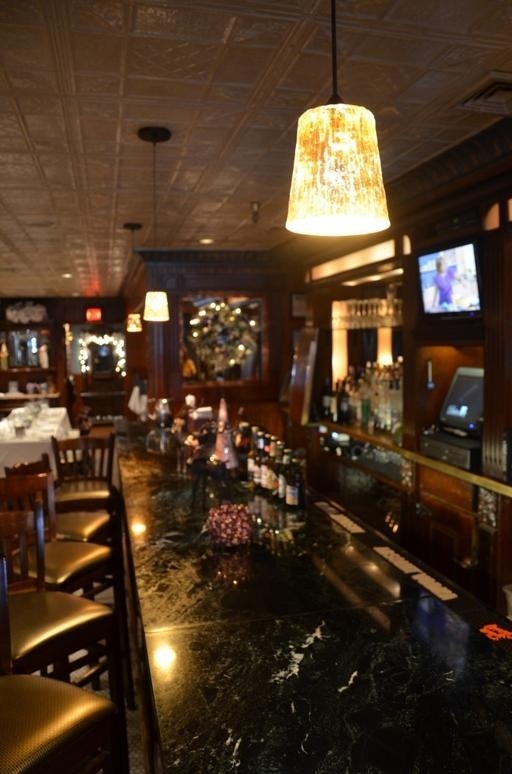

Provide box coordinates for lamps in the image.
[284,17,391,238]
[124,126,172,332]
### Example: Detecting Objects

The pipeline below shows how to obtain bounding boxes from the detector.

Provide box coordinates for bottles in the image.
[246,425,305,510]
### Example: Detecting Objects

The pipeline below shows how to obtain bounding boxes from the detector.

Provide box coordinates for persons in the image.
[430,256,464,310]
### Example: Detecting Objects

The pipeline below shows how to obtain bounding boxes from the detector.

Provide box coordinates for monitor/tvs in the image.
[418,242,480,315]
[441,366,484,432]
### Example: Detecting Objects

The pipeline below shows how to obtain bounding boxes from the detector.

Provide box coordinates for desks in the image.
[1,406,72,481]
